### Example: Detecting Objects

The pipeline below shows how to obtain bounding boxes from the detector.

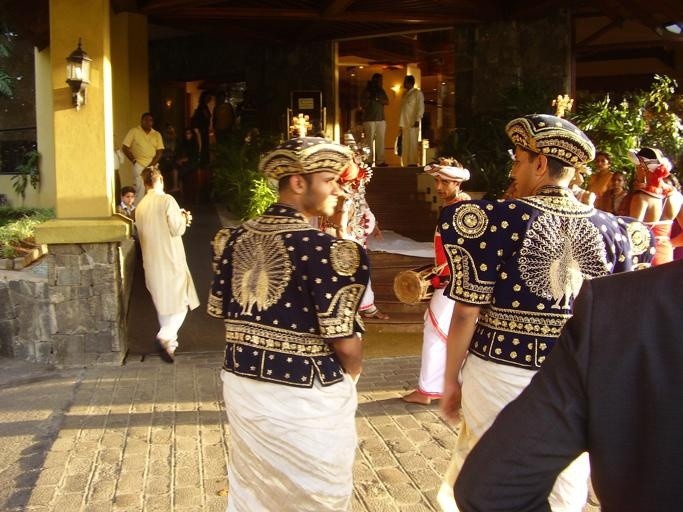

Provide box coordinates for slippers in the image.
[154,338,174,362]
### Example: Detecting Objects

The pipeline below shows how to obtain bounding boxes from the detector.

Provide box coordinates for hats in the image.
[628,145,664,171]
[423,156,470,182]
[258,137,353,180]
[504,114,596,169]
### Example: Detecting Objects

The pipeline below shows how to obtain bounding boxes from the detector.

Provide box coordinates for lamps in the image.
[64,32,95,115]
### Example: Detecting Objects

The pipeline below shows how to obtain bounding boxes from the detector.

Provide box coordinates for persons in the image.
[571,147,682,272]
[321,160,367,333]
[358,72,391,168]
[396,155,474,409]
[349,163,392,324]
[132,162,203,366]
[203,134,373,512]
[432,109,659,512]
[117,85,263,235]
[450,255,683,512]
[396,73,427,169]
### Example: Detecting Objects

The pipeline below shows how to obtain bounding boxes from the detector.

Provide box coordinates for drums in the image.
[394,262,435,305]
[615,215,653,256]
